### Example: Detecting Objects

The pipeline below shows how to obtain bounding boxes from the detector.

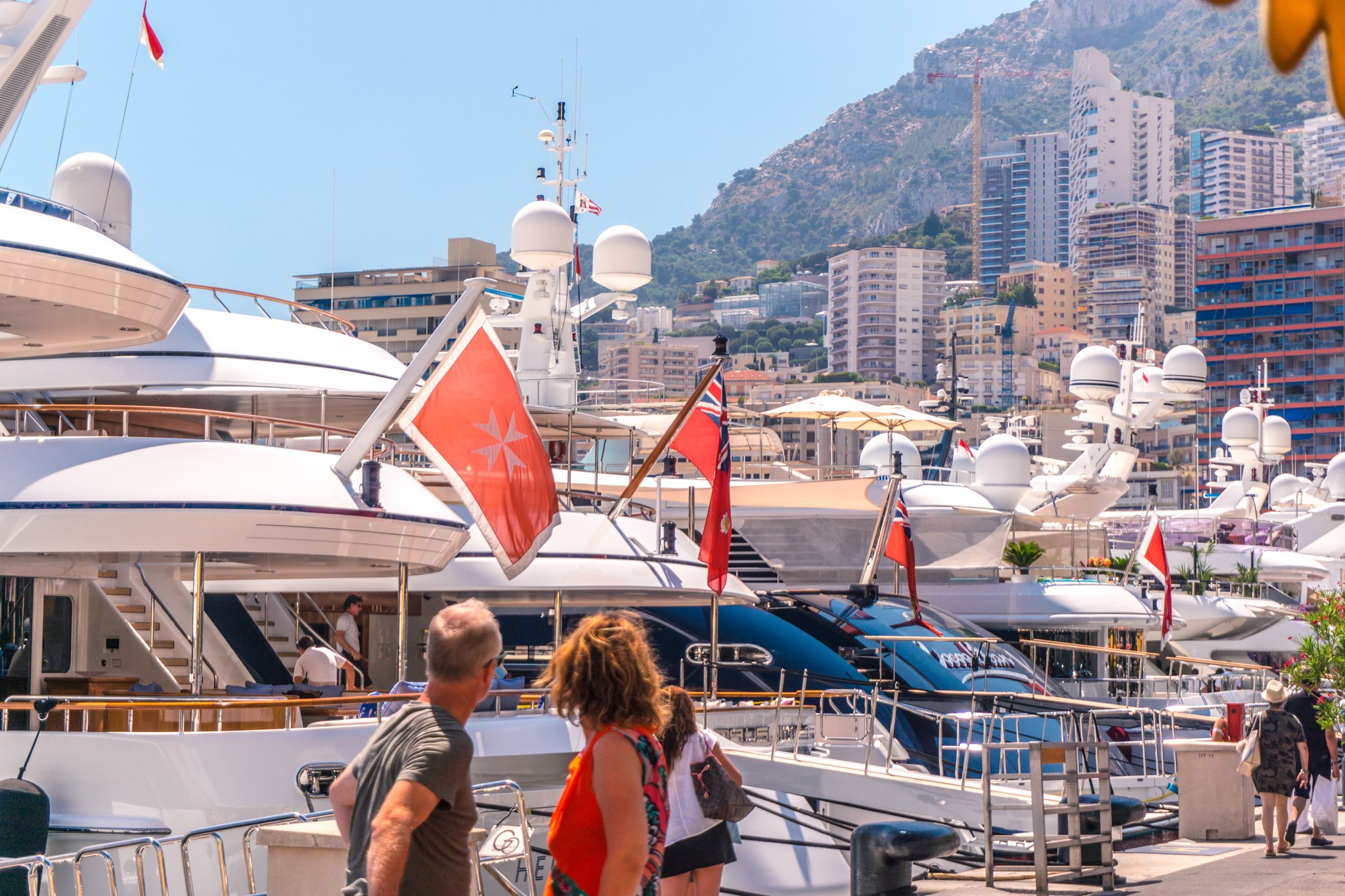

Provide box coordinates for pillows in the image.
[357,676,525,719]
[226,680,345,727]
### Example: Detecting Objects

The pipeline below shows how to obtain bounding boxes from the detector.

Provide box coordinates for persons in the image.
[1113,659,1142,695]
[1186,661,1345,857]
[293,594,744,896]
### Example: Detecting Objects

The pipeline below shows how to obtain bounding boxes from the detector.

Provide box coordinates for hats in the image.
[1261,680,1292,703]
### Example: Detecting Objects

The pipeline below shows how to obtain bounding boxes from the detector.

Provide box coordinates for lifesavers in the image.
[548,439,566,462]
[1208,713,1229,741]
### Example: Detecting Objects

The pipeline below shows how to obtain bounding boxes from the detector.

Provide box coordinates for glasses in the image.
[481,649,507,669]
[354,603,361,608]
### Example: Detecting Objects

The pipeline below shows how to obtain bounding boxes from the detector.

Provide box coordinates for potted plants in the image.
[1064,394,1071,413]
[1021,396,1027,414]
[1002,540,1048,583]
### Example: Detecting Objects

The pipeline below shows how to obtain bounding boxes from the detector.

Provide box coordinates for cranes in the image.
[926,56,1118,300]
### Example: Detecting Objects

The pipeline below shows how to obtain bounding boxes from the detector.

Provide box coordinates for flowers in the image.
[1075,555,1113,578]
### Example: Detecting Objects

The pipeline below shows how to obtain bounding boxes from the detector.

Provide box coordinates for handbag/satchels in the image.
[1310,775,1339,835]
[1236,711,1262,777]
[690,728,755,823]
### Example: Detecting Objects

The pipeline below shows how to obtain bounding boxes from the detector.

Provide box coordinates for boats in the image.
[0,1,1345,896]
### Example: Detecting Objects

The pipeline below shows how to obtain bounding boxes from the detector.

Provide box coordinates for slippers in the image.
[1277,841,1291,852]
[1265,848,1276,856]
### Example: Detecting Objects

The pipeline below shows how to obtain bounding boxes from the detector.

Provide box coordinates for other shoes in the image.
[1285,821,1297,846]
[1310,836,1333,846]
[1299,827,1313,835]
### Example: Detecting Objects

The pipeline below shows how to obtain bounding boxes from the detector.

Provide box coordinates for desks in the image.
[695,705,816,742]
[256,820,487,896]
[44,677,139,732]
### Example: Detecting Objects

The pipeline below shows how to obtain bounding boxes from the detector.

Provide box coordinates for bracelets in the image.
[1301,769,1310,775]
[1332,761,1339,765]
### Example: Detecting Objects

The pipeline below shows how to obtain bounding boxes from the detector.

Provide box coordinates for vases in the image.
[1083,574,1109,582]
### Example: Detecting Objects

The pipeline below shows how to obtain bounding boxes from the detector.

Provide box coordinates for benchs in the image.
[180,689,391,718]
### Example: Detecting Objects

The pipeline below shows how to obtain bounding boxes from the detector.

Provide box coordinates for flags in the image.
[957,439,974,462]
[882,486,923,620]
[137,0,164,71]
[575,189,602,216]
[396,303,561,581]
[575,228,582,285]
[665,361,731,596]
[1137,504,1174,652]
